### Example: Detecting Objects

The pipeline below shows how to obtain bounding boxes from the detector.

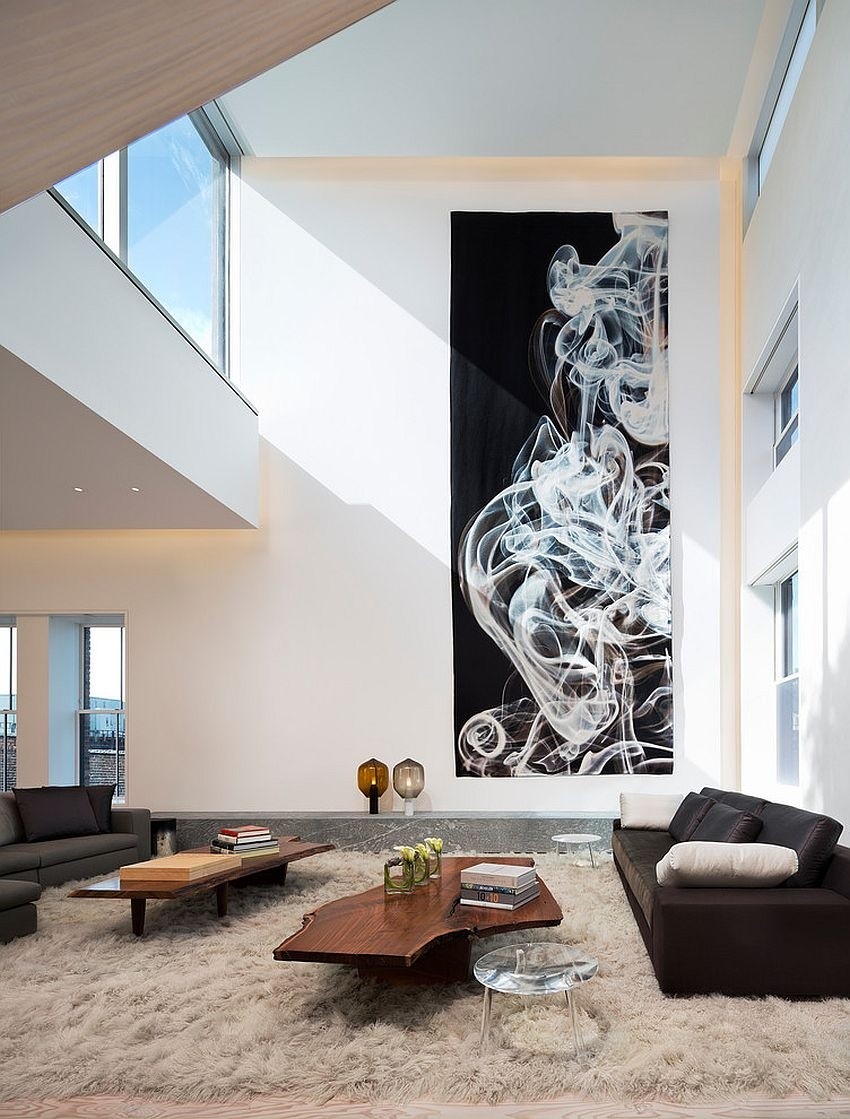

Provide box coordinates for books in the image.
[210,824,280,859]
[459,862,541,911]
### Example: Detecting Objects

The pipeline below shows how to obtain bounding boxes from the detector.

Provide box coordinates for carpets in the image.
[0,852,850,1119]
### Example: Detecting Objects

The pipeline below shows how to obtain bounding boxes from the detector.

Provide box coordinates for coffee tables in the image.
[69,836,335,937]
[272,857,561,993]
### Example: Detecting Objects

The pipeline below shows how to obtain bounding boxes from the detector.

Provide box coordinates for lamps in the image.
[358,758,389,815]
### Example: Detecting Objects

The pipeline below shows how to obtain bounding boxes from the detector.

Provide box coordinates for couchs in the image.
[1,808,152,943]
[613,787,850,1001]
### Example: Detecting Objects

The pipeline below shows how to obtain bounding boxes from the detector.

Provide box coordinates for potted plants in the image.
[384,837,444,895]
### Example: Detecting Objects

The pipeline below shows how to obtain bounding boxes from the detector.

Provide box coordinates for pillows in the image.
[670,791,718,842]
[618,791,684,831]
[86,784,117,833]
[655,840,798,886]
[689,803,763,841]
[13,787,100,842]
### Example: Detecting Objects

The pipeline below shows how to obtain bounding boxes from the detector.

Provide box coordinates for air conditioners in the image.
[394,758,424,816]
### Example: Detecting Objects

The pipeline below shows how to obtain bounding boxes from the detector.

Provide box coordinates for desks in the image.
[551,834,602,869]
[474,942,599,1064]
